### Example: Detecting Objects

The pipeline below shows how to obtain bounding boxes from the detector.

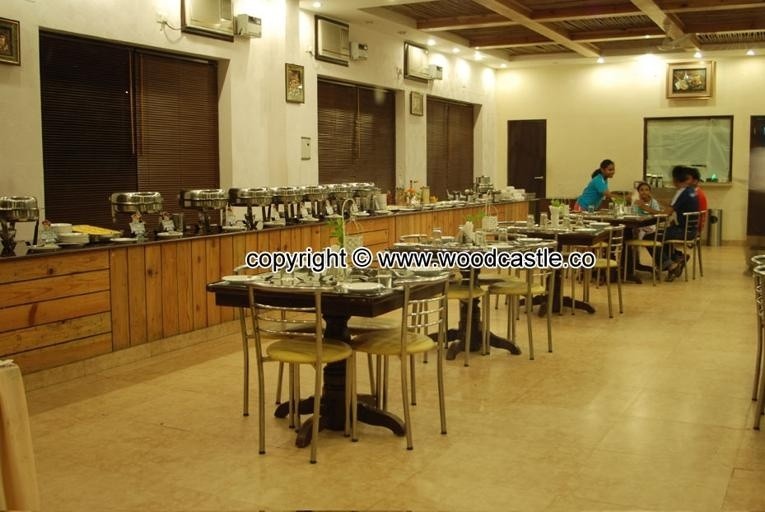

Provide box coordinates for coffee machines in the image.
[474,176,501,203]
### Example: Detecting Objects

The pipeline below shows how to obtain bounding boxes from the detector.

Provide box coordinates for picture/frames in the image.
[409,90,424,116]
[284,62,307,104]
[0,17,21,67]
[665,58,717,100]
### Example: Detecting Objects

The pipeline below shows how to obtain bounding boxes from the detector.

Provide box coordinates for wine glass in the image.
[273,181,376,224]
[0,195,39,253]
[228,185,272,230]
[176,187,228,234]
[110,191,163,240]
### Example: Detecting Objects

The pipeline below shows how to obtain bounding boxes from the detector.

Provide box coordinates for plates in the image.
[222,224,245,231]
[263,221,285,228]
[108,237,139,244]
[515,221,611,236]
[375,210,391,215]
[156,231,184,240]
[501,185,527,203]
[220,266,444,295]
[49,222,91,249]
[391,235,545,254]
[376,199,489,212]
[28,242,61,252]
[580,209,650,217]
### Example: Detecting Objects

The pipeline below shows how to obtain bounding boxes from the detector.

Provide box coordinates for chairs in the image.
[204,191,710,470]
[749,266,765,431]
[751,254,765,401]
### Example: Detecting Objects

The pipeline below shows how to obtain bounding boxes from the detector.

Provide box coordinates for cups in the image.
[373,194,388,210]
[171,213,184,232]
[419,186,431,204]
[429,222,509,251]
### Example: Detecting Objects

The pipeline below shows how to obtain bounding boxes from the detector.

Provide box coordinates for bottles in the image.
[464,189,470,201]
[645,173,665,189]
[407,179,415,194]
[453,191,460,200]
[711,173,717,182]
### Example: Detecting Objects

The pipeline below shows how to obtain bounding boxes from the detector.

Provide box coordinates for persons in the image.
[633,183,661,214]
[643,165,700,282]
[690,168,708,233]
[573,159,633,211]
